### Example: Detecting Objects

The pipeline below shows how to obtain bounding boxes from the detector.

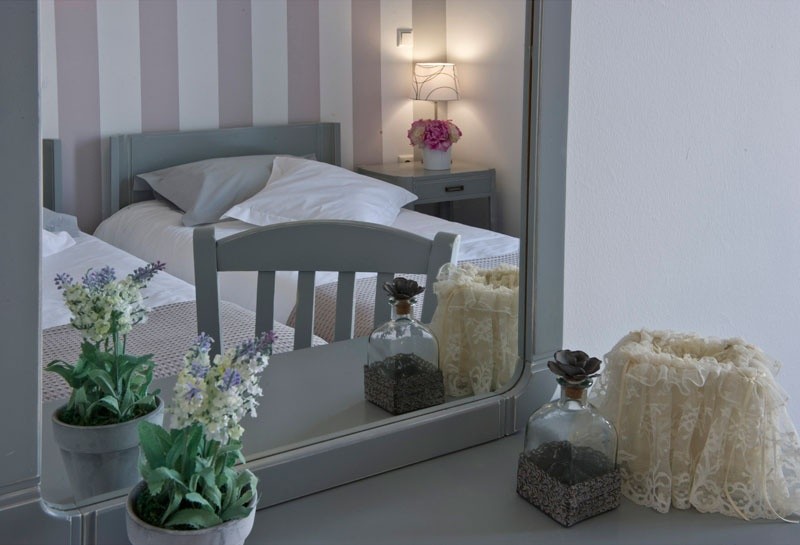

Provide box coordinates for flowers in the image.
[548,349,601,388]
[407,119,462,152]
[136,330,279,529]
[382,277,425,300]
[43,260,167,422]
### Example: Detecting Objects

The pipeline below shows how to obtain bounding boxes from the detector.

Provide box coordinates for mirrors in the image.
[0,1,572,545]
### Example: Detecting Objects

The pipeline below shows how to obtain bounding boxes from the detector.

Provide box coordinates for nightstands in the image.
[355,160,497,232]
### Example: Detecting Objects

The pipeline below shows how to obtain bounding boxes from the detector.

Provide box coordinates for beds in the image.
[41,138,327,402]
[91,122,520,343]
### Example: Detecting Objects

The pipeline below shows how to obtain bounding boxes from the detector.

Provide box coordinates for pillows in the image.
[133,154,316,227]
[219,156,418,226]
[43,207,79,239]
[42,229,75,256]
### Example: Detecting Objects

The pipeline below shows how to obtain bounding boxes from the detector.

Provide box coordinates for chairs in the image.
[192,219,461,366]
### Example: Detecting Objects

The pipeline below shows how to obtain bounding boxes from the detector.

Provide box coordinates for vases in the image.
[423,145,451,170]
[364,298,445,414]
[51,394,163,498]
[516,386,622,528]
[125,477,256,545]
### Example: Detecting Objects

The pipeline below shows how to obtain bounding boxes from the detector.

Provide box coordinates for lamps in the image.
[410,62,461,120]
